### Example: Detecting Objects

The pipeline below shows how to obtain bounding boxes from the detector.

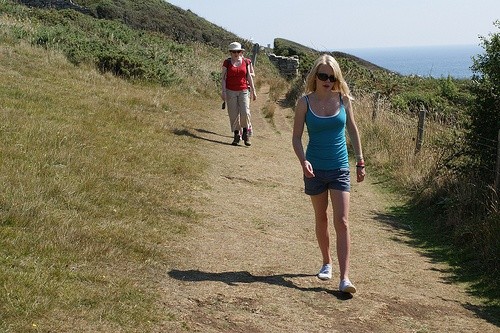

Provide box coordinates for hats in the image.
[229,42,245,51]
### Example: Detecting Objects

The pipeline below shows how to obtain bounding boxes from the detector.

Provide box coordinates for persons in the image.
[221,42,257,146]
[292,54,366,293]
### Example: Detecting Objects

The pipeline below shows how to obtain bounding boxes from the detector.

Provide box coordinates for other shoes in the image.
[240,132,243,140]
[248,128,253,136]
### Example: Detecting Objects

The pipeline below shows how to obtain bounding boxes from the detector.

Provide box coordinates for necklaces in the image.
[234,62,239,66]
[316,91,331,110]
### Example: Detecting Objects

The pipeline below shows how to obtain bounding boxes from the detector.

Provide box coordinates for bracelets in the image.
[353,154,366,170]
[251,88,255,91]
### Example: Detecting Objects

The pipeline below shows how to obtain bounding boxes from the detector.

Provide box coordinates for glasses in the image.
[316,72,337,82]
[232,50,242,53]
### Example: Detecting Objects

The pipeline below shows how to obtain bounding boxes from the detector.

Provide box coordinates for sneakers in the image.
[318,264,332,280]
[232,134,240,146]
[242,133,251,146]
[339,279,356,293]
